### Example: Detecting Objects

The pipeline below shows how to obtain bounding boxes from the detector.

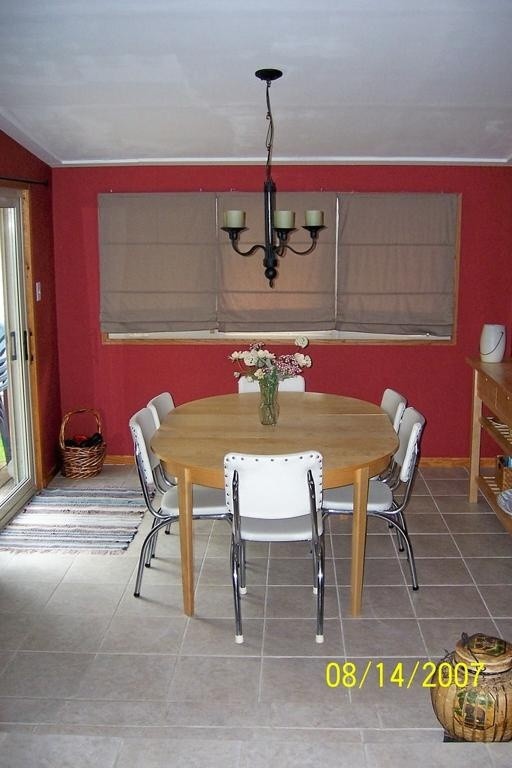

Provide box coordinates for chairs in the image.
[240,372,309,395]
[311,404,428,595]
[0,334,12,464]
[122,403,249,603]
[224,446,330,649]
[143,392,261,594]
[317,387,414,556]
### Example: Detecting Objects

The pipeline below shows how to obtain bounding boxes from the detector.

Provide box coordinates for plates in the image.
[495,485,512,515]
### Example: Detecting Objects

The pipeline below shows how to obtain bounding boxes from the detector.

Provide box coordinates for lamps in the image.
[222,67,329,287]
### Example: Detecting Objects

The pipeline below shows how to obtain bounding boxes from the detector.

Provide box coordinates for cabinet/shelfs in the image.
[465,357,512,537]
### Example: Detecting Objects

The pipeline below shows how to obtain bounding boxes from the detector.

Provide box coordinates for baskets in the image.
[58,407,106,479]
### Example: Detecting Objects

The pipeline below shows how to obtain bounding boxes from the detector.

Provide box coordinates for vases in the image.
[257,378,280,425]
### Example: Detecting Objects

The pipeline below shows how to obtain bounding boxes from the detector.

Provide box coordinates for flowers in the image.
[224,335,316,382]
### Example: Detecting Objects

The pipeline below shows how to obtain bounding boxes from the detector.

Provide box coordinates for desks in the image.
[151,389,400,617]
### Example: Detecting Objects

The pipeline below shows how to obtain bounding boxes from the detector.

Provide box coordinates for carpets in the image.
[0,484,160,562]
